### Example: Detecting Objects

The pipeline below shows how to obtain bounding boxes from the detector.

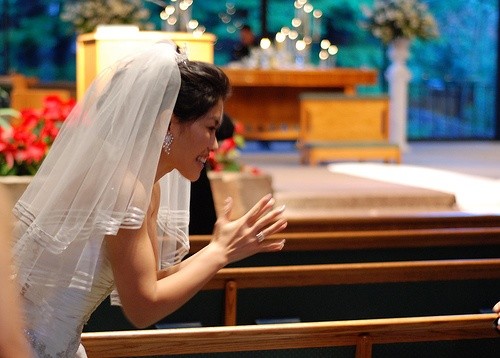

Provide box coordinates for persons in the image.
[9,40,287,358]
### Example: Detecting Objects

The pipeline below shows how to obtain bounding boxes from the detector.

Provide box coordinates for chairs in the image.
[295,94,402,167]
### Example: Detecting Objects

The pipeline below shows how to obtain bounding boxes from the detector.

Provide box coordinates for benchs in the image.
[78,215,500,358]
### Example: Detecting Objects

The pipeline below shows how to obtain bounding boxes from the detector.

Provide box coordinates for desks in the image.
[73,32,216,105]
[219,69,378,142]
[206,167,460,225]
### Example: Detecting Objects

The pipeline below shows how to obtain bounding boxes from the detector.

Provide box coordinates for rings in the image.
[256,233,264,242]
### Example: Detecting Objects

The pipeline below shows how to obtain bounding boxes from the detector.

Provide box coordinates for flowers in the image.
[0,94,77,176]
[59,0,155,34]
[358,0,440,45]
